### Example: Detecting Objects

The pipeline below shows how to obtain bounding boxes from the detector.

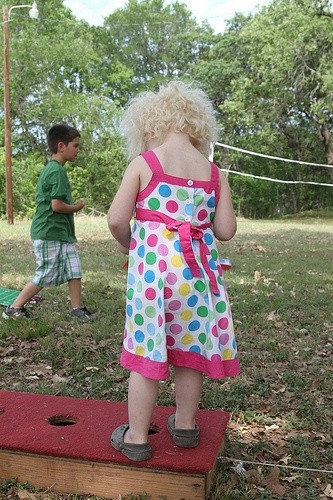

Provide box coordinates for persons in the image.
[1,125,95,322]
[107,82,241,462]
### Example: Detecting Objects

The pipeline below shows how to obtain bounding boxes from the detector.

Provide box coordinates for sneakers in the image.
[1,305,34,321]
[66,306,94,320]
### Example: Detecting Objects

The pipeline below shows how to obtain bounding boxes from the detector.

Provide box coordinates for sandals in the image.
[110,424,150,461]
[166,414,199,447]
